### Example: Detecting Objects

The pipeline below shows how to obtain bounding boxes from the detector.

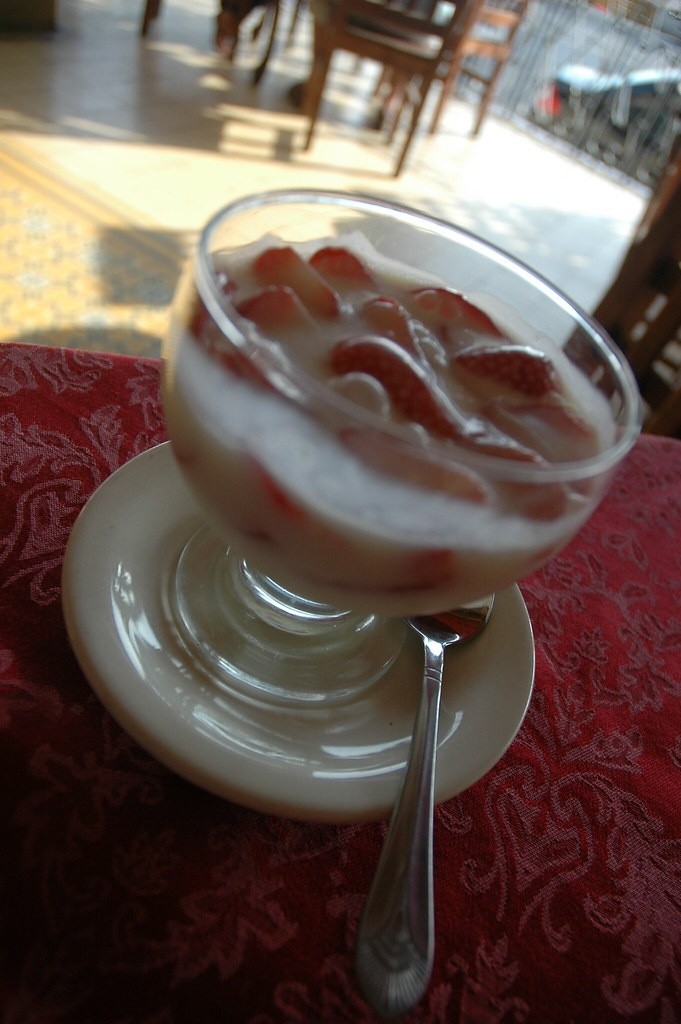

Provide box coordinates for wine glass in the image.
[159,188,645,711]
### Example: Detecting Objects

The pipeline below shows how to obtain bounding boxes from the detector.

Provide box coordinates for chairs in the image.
[304,0,484,178]
[371,0,531,135]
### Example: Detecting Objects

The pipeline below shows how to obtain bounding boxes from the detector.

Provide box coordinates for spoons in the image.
[357,591,497,1018]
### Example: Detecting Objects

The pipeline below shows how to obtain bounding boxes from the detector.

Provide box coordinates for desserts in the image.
[156,238,618,625]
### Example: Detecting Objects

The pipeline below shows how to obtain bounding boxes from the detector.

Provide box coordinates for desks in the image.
[0,343,681,1024]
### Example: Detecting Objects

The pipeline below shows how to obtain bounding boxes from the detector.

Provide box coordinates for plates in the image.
[60,439,535,826]
